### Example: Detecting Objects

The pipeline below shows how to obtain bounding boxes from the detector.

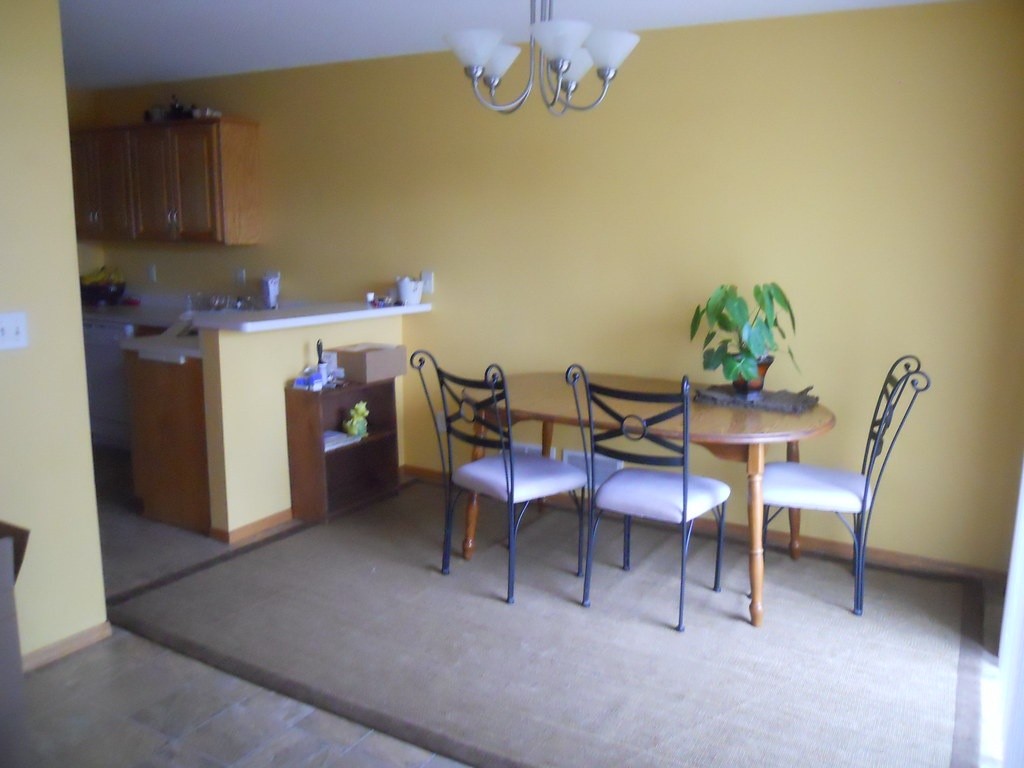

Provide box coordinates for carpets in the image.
[104,476,986,768]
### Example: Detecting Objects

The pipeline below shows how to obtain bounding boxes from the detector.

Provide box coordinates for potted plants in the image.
[690,282,800,393]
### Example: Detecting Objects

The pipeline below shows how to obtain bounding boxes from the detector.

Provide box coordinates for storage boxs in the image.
[324,345,407,384]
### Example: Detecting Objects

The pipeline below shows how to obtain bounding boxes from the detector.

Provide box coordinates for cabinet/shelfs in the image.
[83,310,211,537]
[283,378,400,523]
[70,114,265,246]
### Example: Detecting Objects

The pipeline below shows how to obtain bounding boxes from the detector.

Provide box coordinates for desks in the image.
[461,372,836,627]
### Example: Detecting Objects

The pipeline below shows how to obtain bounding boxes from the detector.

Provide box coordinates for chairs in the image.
[409,349,587,606]
[762,355,930,615]
[566,363,731,632]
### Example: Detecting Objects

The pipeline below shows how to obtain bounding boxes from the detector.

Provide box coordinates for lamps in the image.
[445,0,639,117]
